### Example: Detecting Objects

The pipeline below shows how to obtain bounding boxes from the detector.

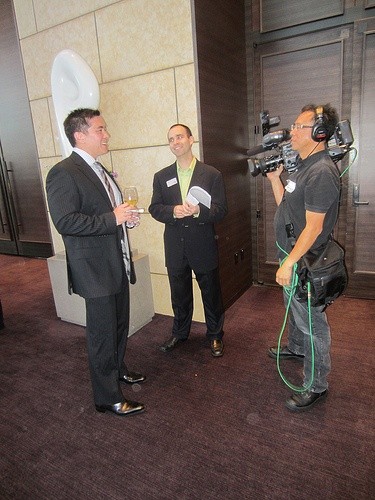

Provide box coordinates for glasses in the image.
[290,123,314,131]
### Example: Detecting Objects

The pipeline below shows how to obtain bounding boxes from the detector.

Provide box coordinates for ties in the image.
[93,162,124,242]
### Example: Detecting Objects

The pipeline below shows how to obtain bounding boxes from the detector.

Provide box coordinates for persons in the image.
[46,109,146,417]
[148,124,227,357]
[265,103,339,411]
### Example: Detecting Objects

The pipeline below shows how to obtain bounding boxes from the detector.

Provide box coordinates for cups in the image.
[123,187,138,207]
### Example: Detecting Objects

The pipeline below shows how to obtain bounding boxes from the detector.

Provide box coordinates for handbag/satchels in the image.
[295,239,347,313]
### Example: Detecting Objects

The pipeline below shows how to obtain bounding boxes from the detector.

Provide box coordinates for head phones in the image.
[311,105,328,142]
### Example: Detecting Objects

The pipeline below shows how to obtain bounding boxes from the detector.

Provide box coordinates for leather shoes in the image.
[284,389,328,412]
[210,336,224,357]
[96,399,146,417]
[268,345,305,362]
[159,336,188,352]
[119,371,148,384]
